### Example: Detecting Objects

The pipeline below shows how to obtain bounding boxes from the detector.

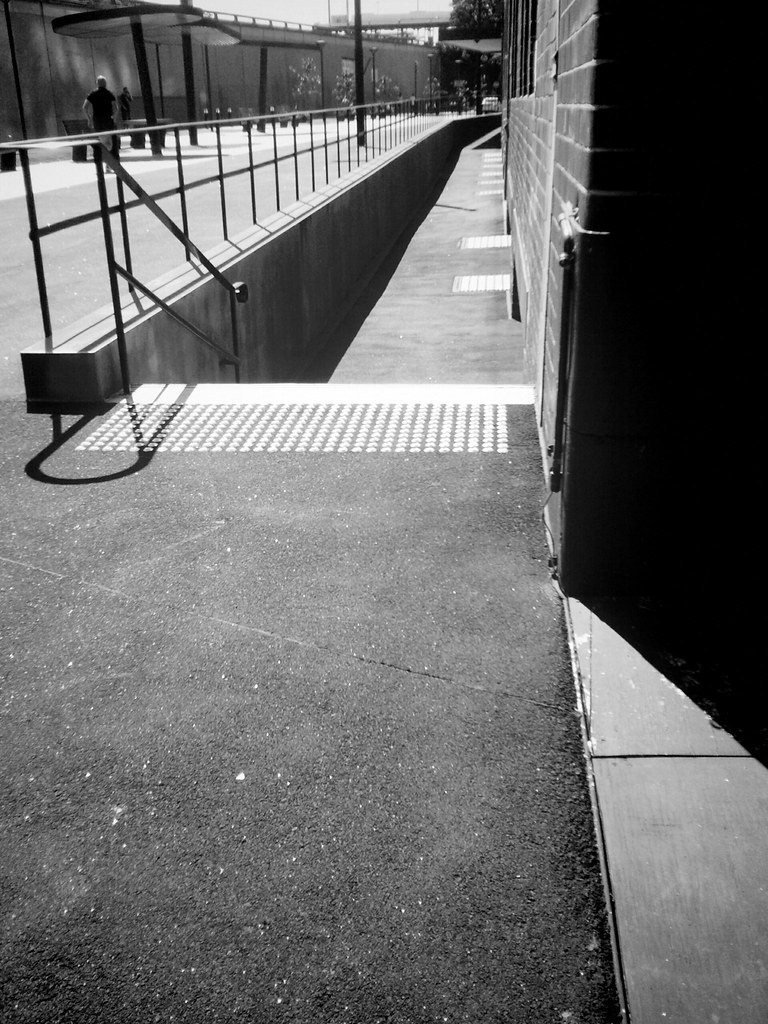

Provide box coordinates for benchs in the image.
[62,119,91,136]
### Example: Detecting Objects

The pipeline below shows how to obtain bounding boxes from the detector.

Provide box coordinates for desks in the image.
[125,118,173,149]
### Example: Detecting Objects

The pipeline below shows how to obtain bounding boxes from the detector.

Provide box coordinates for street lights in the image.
[427,54,434,114]
[315,39,327,109]
[455,60,463,87]
[369,46,378,103]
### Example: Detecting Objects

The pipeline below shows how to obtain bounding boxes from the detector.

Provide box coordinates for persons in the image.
[82,79,120,176]
[118,86,134,128]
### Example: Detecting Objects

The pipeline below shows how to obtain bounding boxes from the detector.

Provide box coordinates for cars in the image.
[480,97,501,114]
